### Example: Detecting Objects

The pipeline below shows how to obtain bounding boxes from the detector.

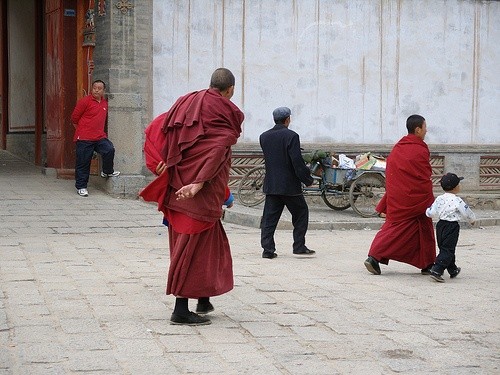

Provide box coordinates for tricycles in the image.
[238,147,387,217]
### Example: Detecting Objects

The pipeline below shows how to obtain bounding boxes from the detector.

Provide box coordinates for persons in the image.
[140,68,244,326]
[365,115,476,283]
[71,80,121,196]
[259,106,316,259]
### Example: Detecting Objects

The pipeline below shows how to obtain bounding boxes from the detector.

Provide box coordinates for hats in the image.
[440,173,464,191]
[273,107,291,120]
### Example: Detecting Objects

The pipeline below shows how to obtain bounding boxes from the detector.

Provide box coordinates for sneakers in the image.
[450,267,461,278]
[293,246,315,256]
[430,272,444,282]
[77,188,88,196]
[101,171,120,178]
[262,253,277,259]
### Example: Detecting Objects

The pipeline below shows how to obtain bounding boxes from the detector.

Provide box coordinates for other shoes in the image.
[196,302,214,314]
[364,257,381,275]
[421,269,431,275]
[169,311,211,326]
